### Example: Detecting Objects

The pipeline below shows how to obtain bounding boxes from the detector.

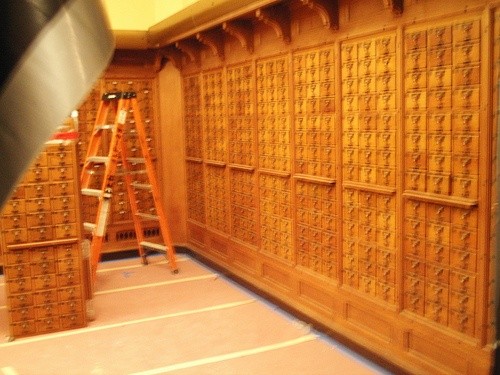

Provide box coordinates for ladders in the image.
[80,90,179,285]
